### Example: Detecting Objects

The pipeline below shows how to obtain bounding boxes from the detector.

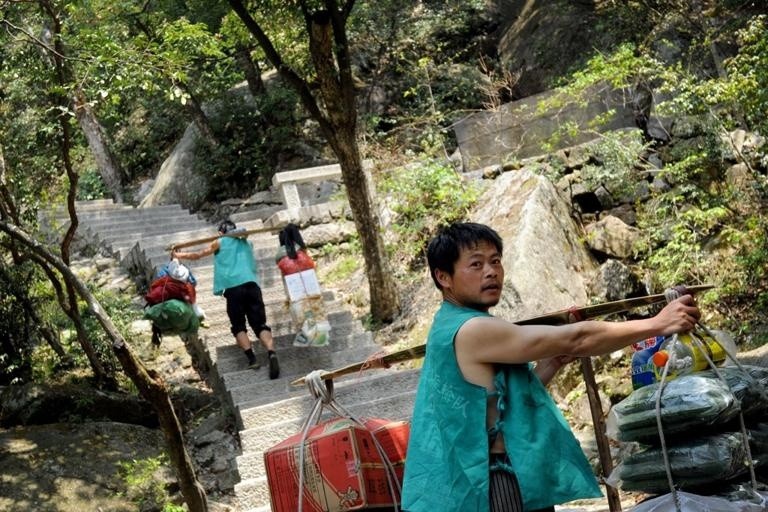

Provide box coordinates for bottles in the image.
[651,329,737,375]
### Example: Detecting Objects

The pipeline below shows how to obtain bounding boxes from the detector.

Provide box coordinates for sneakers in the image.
[247,362,261,369]
[270,354,280,379]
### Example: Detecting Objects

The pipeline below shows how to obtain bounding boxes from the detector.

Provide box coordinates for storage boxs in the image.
[284,269,320,298]
[262,412,410,512]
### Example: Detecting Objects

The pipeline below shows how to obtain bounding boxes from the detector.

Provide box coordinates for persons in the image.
[169,221,280,380]
[400,222,699,511]
